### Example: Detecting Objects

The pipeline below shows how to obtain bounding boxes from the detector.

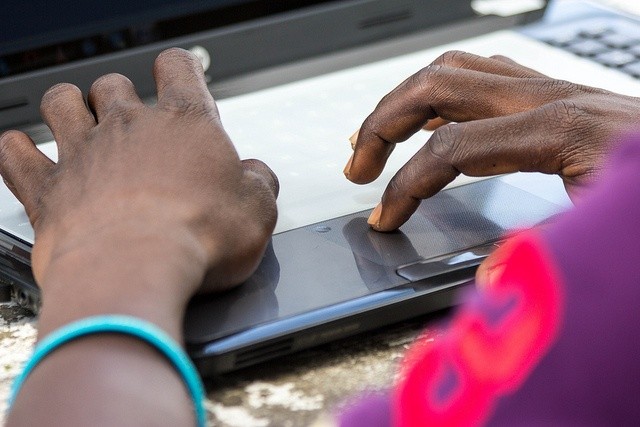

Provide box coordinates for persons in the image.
[1,46,640,426]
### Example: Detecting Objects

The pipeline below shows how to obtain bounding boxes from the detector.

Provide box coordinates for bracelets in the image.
[8,313,208,425]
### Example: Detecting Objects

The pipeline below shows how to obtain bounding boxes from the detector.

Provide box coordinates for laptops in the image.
[1,1,637,385]
